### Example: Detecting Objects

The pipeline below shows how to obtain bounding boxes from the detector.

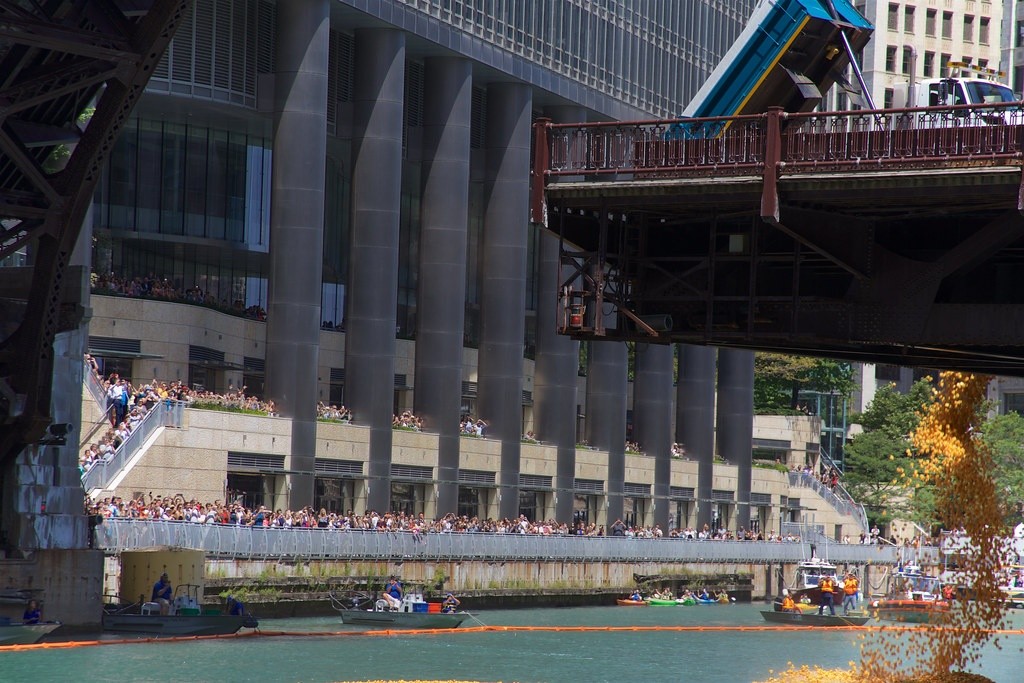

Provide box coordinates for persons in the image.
[80,351,277,469]
[91,268,267,321]
[579,439,588,446]
[322,320,344,329]
[843,525,896,546]
[85,490,429,535]
[903,526,967,548]
[629,585,726,602]
[818,574,836,616]
[609,517,801,543]
[625,440,640,452]
[151,573,174,616]
[22,601,40,625]
[796,402,810,416]
[842,572,861,616]
[782,593,814,614]
[526,431,535,440]
[775,459,840,494]
[993,569,1024,590]
[671,443,687,459]
[442,592,461,613]
[942,584,952,604]
[383,575,402,610]
[317,400,488,439]
[429,512,604,536]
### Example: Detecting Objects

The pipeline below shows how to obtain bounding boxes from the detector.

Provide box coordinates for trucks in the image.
[668,0,1024,146]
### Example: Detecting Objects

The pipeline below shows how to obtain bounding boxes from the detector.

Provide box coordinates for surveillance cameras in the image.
[50,423,73,435]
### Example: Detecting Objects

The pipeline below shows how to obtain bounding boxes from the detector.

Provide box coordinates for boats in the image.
[837,575,863,602]
[103,583,264,639]
[329,582,481,629]
[760,609,871,626]
[719,597,729,605]
[785,515,846,606]
[866,542,952,625]
[644,596,696,606]
[616,598,651,606]
[939,562,1024,609]
[691,593,718,603]
